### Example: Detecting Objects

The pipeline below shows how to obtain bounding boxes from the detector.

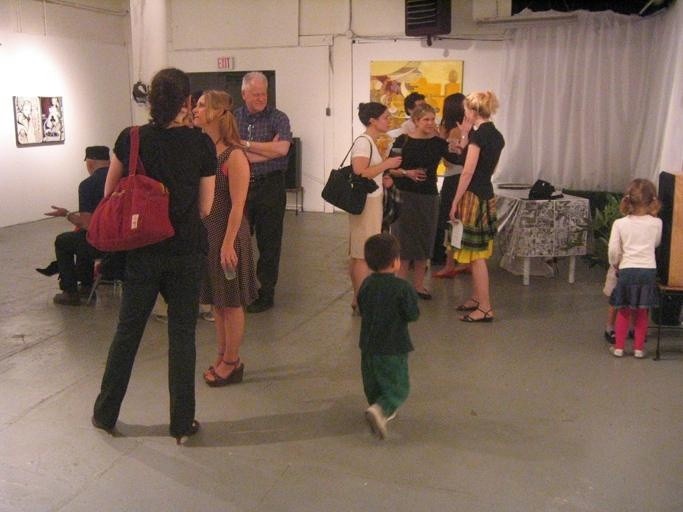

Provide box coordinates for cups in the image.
[220,260,237,280]
[391,148,402,159]
[417,167,428,182]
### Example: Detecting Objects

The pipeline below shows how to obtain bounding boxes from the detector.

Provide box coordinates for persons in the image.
[233,72,291,313]
[348,90,505,322]
[192,90,260,387]
[37,145,120,306]
[603,266,635,343]
[601,177,664,359]
[92,68,218,444]
[356,233,421,441]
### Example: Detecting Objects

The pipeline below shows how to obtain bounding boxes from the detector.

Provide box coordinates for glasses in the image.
[248,123,255,141]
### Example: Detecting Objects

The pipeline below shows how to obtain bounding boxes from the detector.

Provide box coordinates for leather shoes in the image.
[36,262,96,305]
[416,285,432,301]
[431,267,472,278]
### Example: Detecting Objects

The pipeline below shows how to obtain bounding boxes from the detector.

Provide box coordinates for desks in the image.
[285,187,304,217]
[490,180,589,288]
[652,283,683,362]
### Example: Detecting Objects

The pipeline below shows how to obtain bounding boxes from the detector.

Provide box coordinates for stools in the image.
[83,267,127,303]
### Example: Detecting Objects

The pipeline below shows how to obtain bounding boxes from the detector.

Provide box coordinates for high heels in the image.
[175,421,198,445]
[205,358,244,387]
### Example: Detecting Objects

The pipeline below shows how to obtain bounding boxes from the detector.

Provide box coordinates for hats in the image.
[83,146,109,162]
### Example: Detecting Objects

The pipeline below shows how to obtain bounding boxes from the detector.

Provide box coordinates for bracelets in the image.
[246,142,250,150]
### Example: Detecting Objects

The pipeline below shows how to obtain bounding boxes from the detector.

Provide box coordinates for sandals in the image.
[459,307,493,321]
[456,300,479,312]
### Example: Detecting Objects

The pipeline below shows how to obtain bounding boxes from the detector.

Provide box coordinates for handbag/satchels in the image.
[529,179,564,200]
[85,176,175,252]
[321,169,366,214]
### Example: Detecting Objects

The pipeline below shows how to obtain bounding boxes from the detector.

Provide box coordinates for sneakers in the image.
[247,295,274,313]
[606,328,644,359]
[364,402,401,442]
